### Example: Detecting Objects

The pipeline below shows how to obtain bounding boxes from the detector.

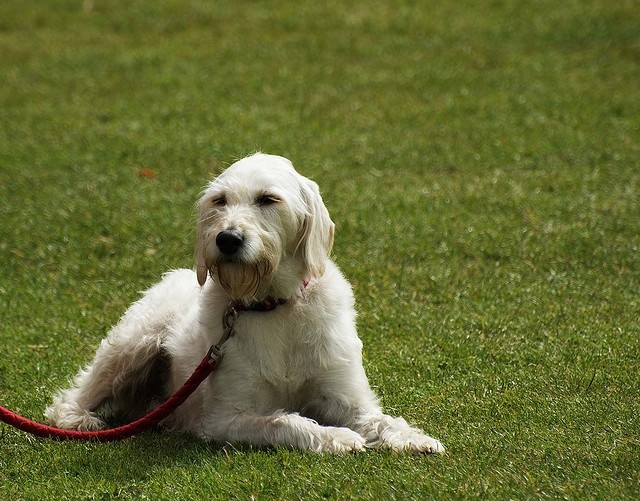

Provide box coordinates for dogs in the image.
[42,151,447,458]
